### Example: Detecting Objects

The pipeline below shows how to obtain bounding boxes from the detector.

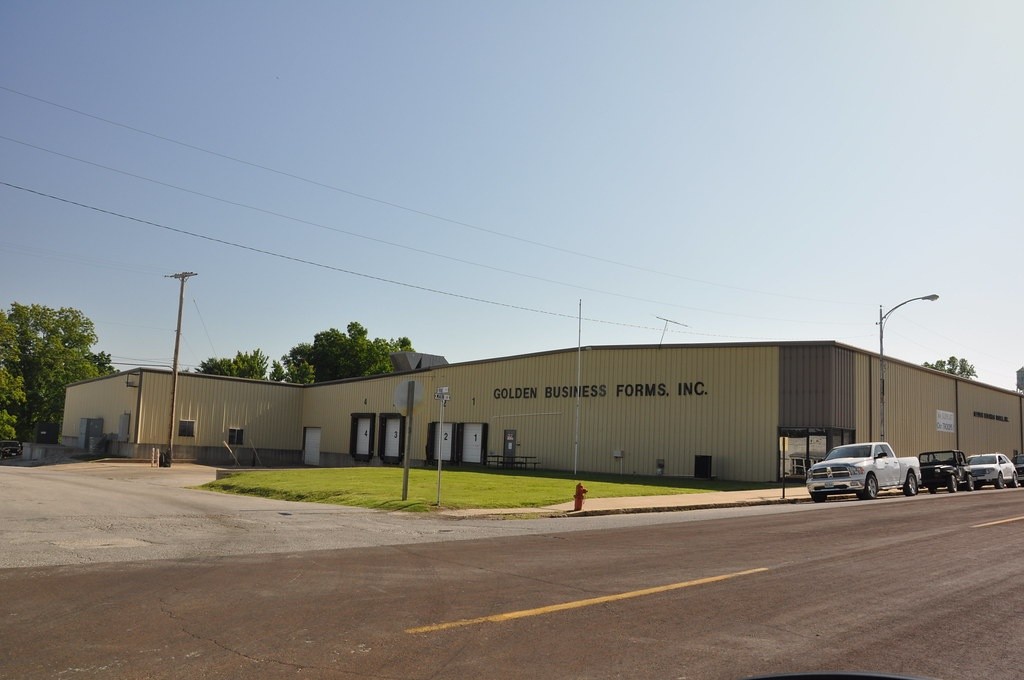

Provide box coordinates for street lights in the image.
[877,293,940,442]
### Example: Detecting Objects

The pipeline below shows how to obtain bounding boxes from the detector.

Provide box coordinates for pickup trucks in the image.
[804,442,923,502]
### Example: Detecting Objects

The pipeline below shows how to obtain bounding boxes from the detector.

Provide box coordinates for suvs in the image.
[915,449,974,494]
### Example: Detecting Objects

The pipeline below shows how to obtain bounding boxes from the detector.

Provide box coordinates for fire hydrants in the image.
[574,483,588,511]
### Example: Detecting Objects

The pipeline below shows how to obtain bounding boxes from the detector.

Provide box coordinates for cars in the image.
[1009,454,1024,485]
[0,440,22,458]
[962,454,1018,489]
